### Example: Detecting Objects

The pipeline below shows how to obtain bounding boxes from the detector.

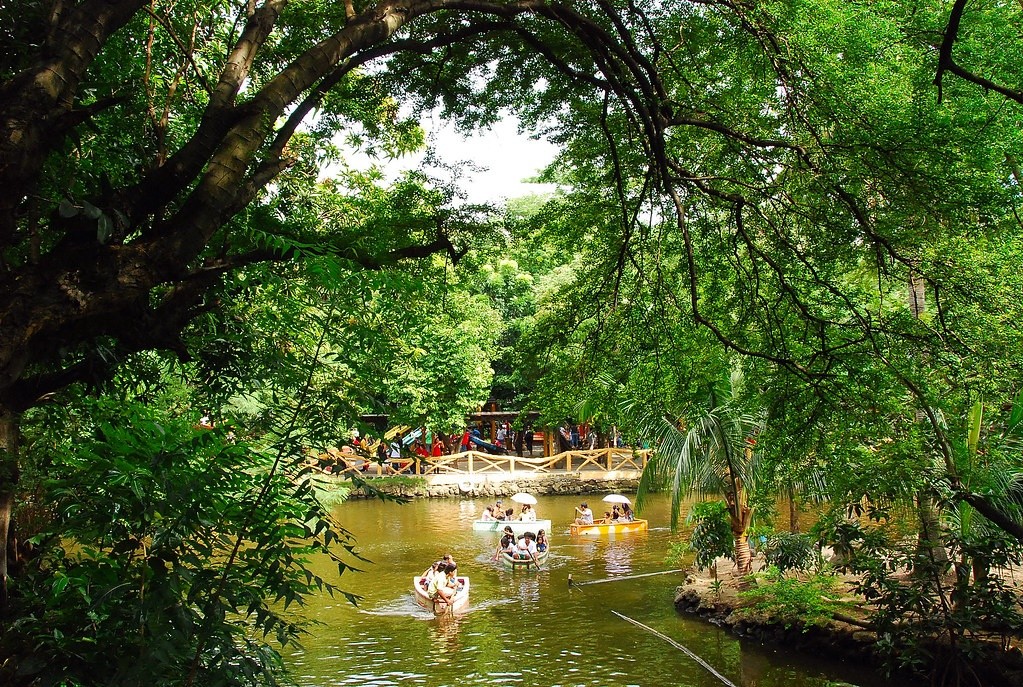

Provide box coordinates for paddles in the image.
[527,549,540,570]
[575,509,578,523]
[442,586,458,617]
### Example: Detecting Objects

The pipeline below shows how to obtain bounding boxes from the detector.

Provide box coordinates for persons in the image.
[575,503,633,525]
[419,554,460,606]
[310,405,757,475]
[482,500,536,521]
[199,413,213,427]
[493,525,549,561]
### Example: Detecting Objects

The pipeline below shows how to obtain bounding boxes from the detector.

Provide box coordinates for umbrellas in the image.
[510,493,538,504]
[602,494,632,514]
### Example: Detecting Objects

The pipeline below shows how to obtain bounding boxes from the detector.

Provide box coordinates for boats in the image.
[569,517,648,536]
[495,543,550,571]
[413,576,470,618]
[472,517,552,534]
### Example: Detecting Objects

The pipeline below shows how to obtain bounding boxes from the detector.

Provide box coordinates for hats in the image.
[497,500,502,504]
